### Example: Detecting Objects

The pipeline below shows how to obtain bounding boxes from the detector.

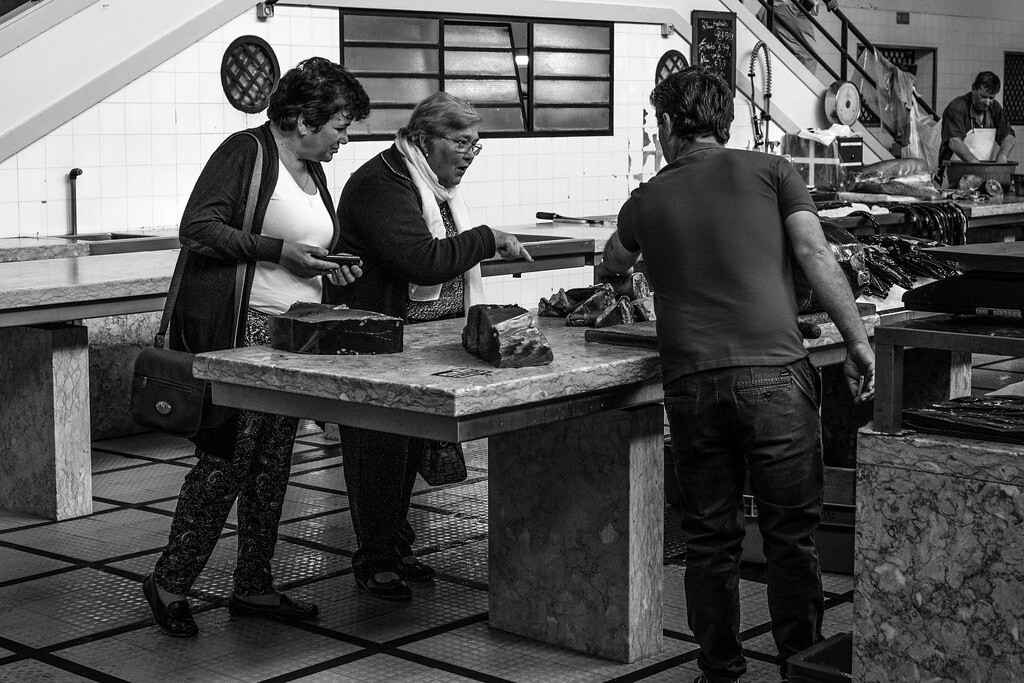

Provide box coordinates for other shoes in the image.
[228,589,318,623]
[380,556,435,579]
[144,572,199,637]
[694,670,741,683]
[354,567,412,600]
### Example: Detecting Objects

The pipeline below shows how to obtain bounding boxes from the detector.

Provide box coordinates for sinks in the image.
[56,231,161,245]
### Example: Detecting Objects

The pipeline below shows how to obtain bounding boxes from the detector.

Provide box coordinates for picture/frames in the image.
[693,10,737,98]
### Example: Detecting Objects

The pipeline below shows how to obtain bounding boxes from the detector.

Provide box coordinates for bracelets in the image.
[600,257,620,276]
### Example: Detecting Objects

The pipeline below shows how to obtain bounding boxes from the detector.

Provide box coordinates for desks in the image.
[0,195,1024,683]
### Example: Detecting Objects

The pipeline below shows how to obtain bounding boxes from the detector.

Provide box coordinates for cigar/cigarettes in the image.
[857,374,865,395]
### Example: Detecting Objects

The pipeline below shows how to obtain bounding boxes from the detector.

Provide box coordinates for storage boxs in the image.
[784,631,852,682]
[741,466,856,572]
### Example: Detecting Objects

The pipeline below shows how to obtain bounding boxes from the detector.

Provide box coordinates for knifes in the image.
[535,211,601,222]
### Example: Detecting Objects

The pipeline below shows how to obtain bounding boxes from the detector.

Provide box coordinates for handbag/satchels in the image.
[131,346,226,438]
[416,437,468,486]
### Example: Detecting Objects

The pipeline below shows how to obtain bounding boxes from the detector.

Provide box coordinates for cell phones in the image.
[310,254,360,268]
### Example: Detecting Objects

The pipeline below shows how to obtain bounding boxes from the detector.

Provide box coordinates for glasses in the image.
[432,132,483,157]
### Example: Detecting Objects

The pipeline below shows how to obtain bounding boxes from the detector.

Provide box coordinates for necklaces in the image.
[279,154,308,191]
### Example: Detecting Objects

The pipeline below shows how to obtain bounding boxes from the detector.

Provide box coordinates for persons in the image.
[938,71,1016,190]
[144,57,371,636]
[597,65,876,683]
[335,91,535,600]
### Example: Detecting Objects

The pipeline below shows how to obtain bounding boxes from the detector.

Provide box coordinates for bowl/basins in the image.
[946,159,1019,197]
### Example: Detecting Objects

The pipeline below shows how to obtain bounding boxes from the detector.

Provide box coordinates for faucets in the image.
[69,167,83,234]
